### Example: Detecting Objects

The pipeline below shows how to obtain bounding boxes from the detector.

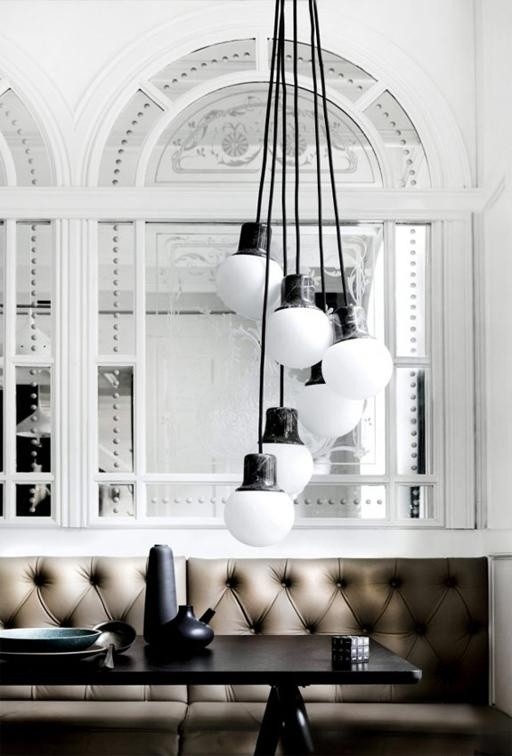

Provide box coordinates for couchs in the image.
[179,556,511,755]
[1,556,187,754]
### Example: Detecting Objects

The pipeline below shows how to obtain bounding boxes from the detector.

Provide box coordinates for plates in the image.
[0,627,106,658]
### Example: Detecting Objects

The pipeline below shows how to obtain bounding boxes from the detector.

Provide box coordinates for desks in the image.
[1,631,423,754]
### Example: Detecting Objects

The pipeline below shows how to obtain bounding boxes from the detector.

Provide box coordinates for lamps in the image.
[216,0,393,547]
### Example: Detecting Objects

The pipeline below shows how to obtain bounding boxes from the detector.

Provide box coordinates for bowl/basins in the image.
[96,620,137,667]
